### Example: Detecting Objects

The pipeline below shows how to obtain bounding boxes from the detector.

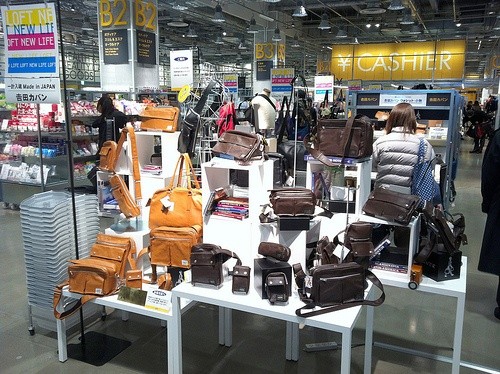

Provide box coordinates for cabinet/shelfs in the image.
[0,90,467,374]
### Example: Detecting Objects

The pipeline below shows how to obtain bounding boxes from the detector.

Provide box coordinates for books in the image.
[371,262,408,274]
[311,165,344,201]
[203,186,249,221]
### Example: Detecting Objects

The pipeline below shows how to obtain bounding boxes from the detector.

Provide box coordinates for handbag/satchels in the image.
[108,174,141,219]
[466,124,485,139]
[410,139,443,210]
[98,140,119,172]
[267,151,290,186]
[315,117,374,158]
[177,124,196,158]
[211,130,261,163]
[276,140,310,172]
[149,187,203,270]
[190,186,465,309]
[89,234,131,279]
[66,259,117,297]
[140,107,179,133]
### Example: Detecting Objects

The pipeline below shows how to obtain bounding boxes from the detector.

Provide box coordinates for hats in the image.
[263,87,271,93]
[296,90,305,99]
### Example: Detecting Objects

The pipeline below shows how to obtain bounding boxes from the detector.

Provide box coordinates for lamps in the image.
[247,20,258,33]
[292,0,308,17]
[336,25,347,38]
[172,0,188,10]
[166,0,461,41]
[272,28,281,41]
[74,40,84,50]
[235,51,243,60]
[210,5,226,22]
[387,0,500,42]
[81,16,95,31]
[214,47,223,55]
[290,36,300,47]
[215,36,225,45]
[318,13,331,29]
[70,5,75,12]
[167,16,188,27]
[238,39,247,50]
[349,35,359,45]
[186,24,198,38]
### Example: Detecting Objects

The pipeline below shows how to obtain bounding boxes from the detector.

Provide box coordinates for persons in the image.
[217,99,235,136]
[87,96,120,187]
[251,88,276,129]
[463,101,500,320]
[371,103,436,247]
[287,90,346,140]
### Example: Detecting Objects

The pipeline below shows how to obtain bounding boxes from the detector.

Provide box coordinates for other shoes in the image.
[480,151,482,153]
[469,150,480,153]
